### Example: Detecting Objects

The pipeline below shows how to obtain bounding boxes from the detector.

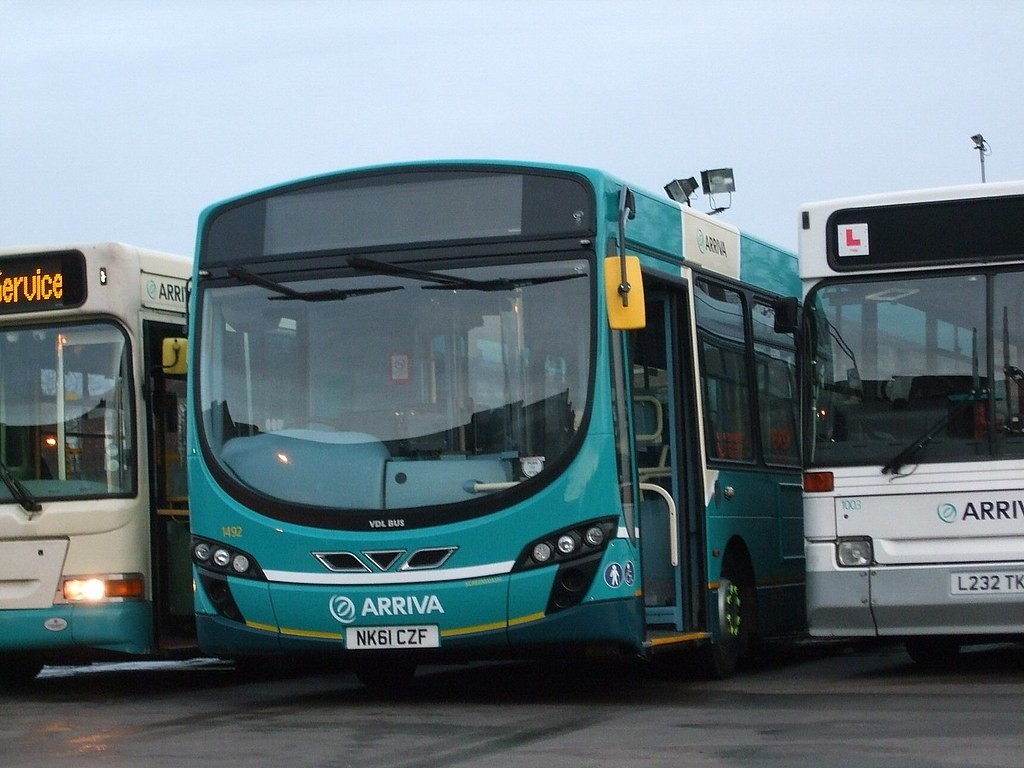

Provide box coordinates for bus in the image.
[772,182,1024,670]
[0,242,577,690]
[180,155,1022,690]
